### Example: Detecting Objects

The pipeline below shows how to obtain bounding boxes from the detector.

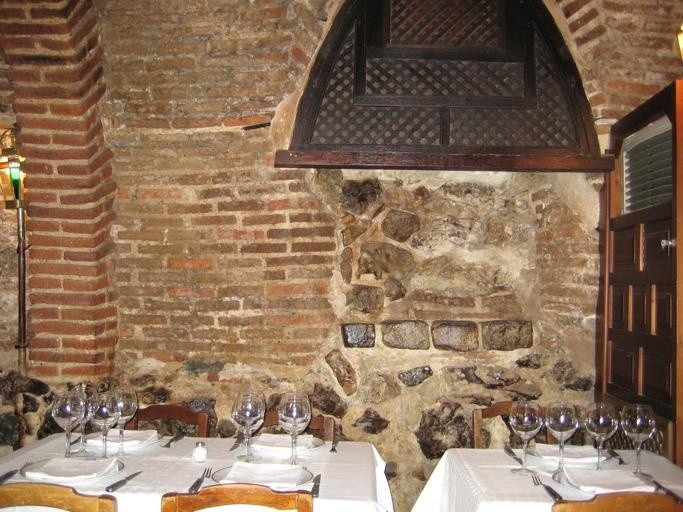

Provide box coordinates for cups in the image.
[192,442,207,463]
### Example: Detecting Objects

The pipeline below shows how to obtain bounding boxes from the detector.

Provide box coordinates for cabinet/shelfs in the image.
[599,205,680,464]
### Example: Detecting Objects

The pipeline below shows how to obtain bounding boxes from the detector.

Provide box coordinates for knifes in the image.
[70,436,80,445]
[311,474,320,497]
[106,471,141,493]
[632,471,679,504]
[503,447,523,466]
[229,439,241,451]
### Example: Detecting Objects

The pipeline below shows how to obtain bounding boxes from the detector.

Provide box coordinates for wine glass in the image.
[584,402,618,472]
[51,387,137,460]
[508,401,543,476]
[620,405,654,480]
[230,393,311,466]
[544,403,578,475]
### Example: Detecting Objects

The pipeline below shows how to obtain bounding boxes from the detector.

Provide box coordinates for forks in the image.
[188,468,211,493]
[159,435,183,448]
[0,462,33,483]
[329,440,337,454]
[530,474,562,502]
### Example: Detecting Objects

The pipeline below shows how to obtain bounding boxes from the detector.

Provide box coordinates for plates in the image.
[19,459,124,481]
[524,446,659,494]
[81,433,161,446]
[244,436,325,451]
[212,466,313,488]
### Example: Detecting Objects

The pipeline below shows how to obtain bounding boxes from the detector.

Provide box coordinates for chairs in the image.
[158,479,315,511]
[1,480,121,511]
[471,397,580,448]
[127,403,211,436]
[250,412,334,444]
[550,488,683,511]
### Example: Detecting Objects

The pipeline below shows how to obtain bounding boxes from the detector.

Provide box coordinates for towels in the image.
[219,457,301,492]
[535,442,611,464]
[561,463,649,498]
[25,452,118,479]
[247,431,318,460]
[89,423,157,453]
